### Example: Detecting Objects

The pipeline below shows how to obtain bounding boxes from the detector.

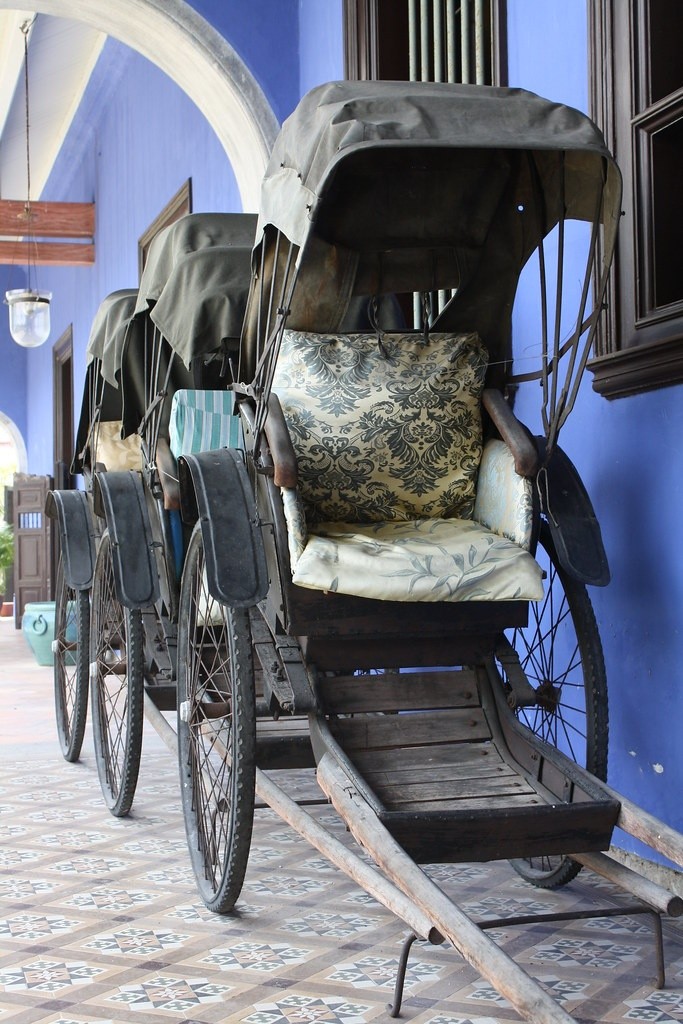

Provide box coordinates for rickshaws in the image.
[49,80,683,1024]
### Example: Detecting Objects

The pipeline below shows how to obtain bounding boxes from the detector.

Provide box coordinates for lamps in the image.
[3,17,54,349]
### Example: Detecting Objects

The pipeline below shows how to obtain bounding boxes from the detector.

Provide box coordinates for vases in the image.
[22,600,97,666]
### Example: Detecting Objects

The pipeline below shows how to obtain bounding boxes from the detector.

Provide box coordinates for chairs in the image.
[264,328,544,602]
[154,389,243,508]
[90,420,143,470]
